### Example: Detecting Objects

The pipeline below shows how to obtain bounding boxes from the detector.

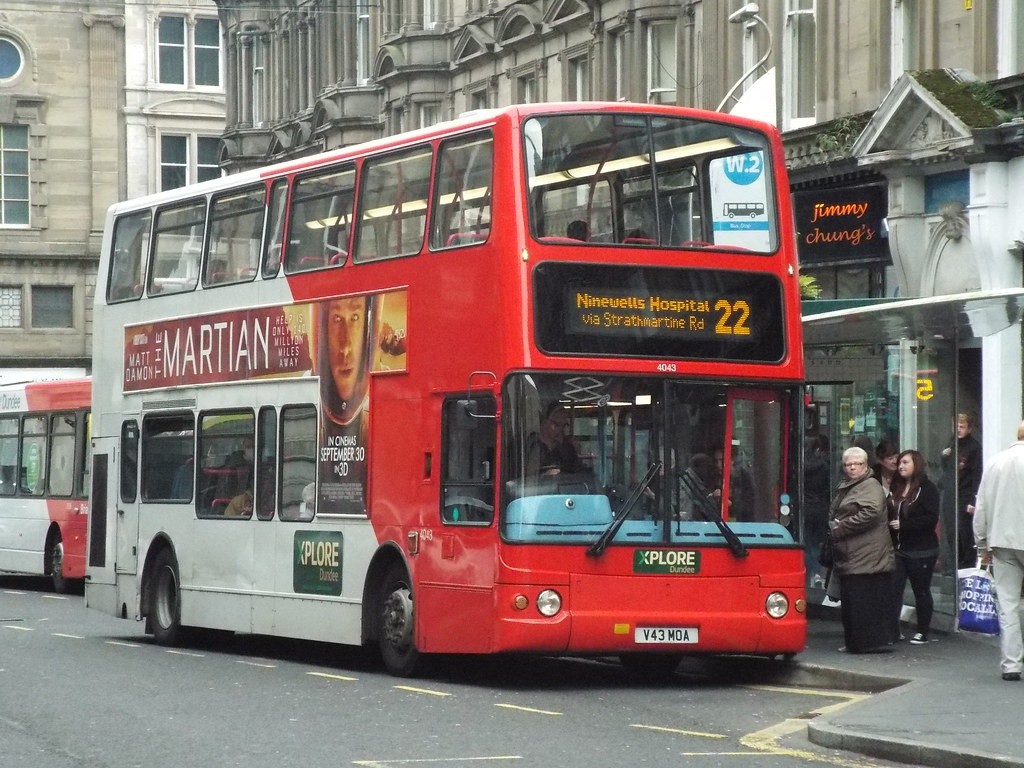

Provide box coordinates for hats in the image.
[689,452,714,466]
[851,434,873,449]
[543,399,565,427]
[876,440,899,458]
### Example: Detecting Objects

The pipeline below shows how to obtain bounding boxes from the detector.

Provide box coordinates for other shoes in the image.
[1002,673,1021,681]
[837,645,848,652]
[941,569,955,576]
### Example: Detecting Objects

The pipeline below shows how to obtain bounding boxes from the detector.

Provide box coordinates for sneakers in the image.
[909,633,929,645]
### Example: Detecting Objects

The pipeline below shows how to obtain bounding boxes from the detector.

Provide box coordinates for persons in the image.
[566,221,592,242]
[326,297,369,407]
[224,479,275,517]
[673,438,755,524]
[829,410,1024,680]
[525,409,594,477]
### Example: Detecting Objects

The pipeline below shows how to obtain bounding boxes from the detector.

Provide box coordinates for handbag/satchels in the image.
[817,537,833,569]
[956,558,999,637]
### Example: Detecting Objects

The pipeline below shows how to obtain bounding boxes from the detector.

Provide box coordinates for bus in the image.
[83,97,809,679]
[0,378,95,594]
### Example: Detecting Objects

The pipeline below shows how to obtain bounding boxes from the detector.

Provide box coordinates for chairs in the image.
[113,231,717,298]
[145,486,275,519]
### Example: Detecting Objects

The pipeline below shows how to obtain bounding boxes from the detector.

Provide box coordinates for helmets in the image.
[307,288,383,426]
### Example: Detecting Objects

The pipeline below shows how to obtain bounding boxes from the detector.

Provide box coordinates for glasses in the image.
[843,461,864,468]
